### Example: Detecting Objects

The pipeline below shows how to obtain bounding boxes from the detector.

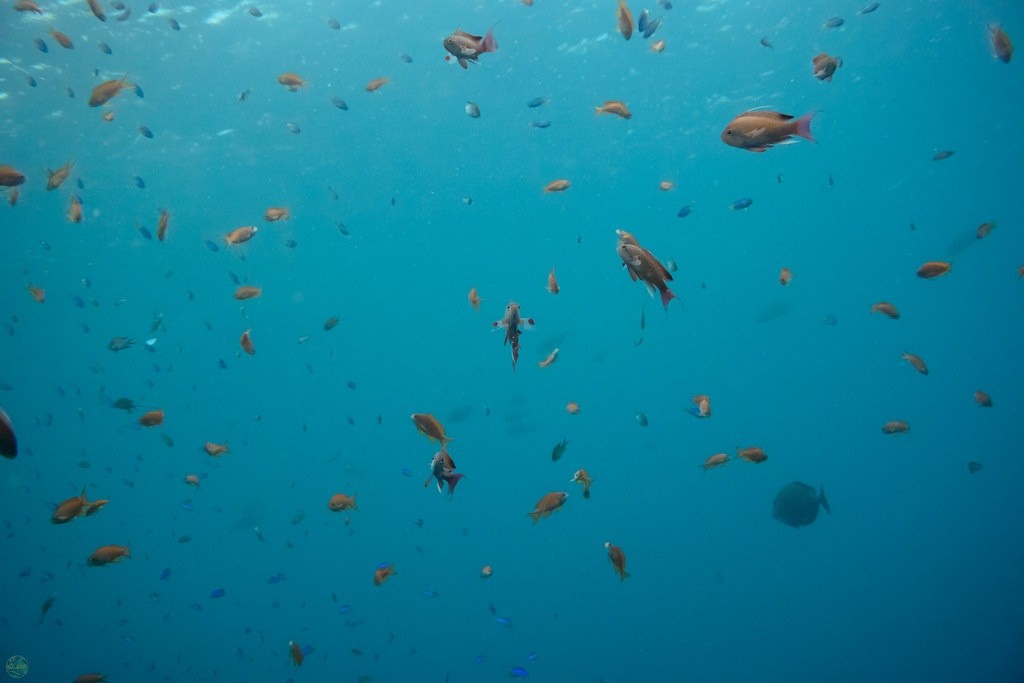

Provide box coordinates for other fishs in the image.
[0,2,1023,683]
